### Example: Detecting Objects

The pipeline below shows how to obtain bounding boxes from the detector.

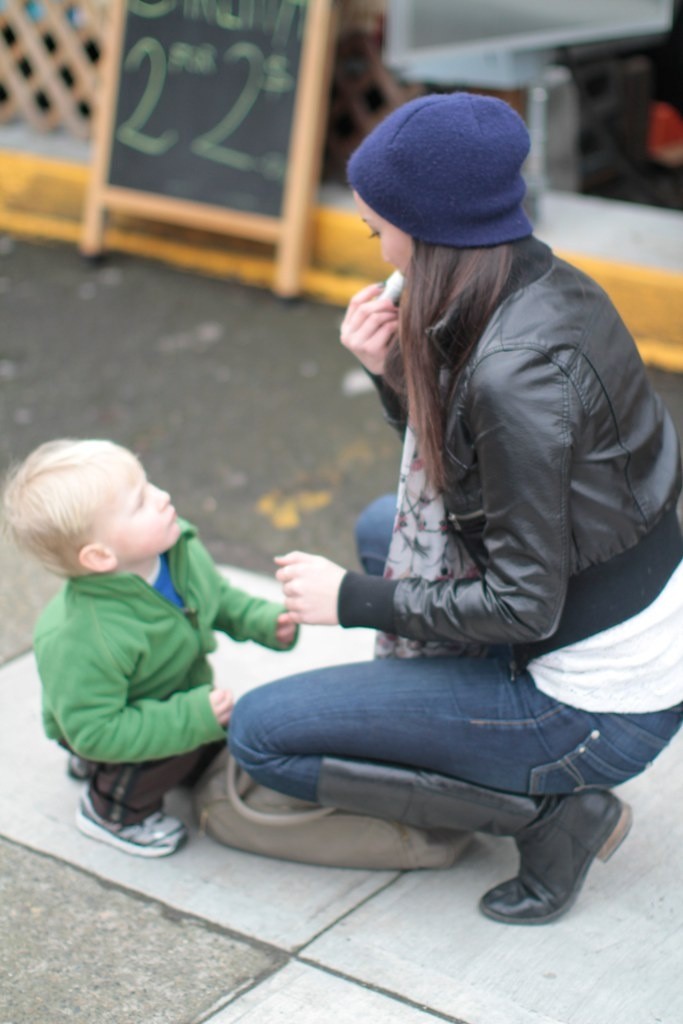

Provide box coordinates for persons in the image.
[227,92,683,924]
[5,438,300,857]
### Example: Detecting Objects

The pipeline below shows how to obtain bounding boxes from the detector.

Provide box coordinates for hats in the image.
[347,90,533,248]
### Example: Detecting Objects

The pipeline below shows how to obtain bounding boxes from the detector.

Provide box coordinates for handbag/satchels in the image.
[195,745,472,868]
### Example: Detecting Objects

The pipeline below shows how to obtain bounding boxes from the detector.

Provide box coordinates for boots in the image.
[318,755,628,923]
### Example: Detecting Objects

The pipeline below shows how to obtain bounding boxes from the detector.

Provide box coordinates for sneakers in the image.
[75,789,185,859]
[68,755,94,778]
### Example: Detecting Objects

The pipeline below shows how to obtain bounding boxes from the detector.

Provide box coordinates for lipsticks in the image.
[372,271,405,305]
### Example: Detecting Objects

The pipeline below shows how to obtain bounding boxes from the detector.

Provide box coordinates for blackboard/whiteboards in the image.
[81,0,348,235]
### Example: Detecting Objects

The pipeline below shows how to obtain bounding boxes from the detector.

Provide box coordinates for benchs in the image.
[379,0,675,192]
